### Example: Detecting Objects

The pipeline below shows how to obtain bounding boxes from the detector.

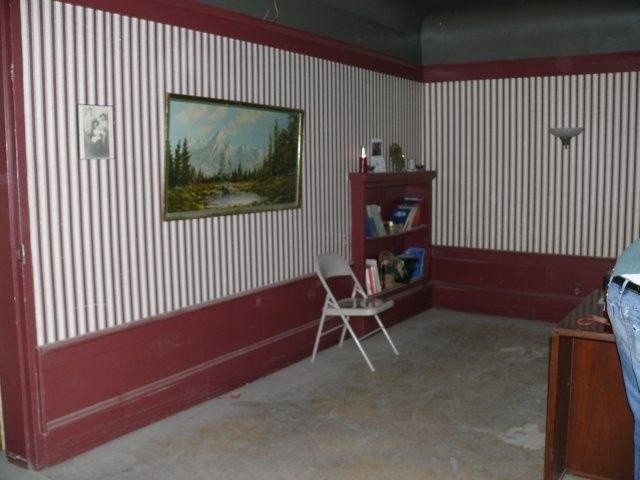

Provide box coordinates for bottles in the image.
[359,147,367,174]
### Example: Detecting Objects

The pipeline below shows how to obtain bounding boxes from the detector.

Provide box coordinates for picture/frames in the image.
[160,88,307,223]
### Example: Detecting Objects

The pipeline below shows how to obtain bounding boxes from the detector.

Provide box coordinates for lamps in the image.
[548,124,587,148]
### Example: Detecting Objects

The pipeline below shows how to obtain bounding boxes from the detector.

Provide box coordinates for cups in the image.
[408,160,415,171]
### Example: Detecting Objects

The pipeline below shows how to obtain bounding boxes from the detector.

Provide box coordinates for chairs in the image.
[309,250,400,372]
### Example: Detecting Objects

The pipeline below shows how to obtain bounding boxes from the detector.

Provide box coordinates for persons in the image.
[602,233,640,479]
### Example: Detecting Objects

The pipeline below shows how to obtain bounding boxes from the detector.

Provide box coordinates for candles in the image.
[359,145,369,172]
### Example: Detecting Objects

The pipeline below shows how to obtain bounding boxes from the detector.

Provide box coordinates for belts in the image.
[611,276,640,296]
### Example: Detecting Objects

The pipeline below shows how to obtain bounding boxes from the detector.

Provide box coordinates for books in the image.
[363,246,427,297]
[364,194,424,236]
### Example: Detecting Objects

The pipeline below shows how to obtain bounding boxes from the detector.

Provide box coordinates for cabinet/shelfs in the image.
[541,286,635,479]
[347,166,441,337]
